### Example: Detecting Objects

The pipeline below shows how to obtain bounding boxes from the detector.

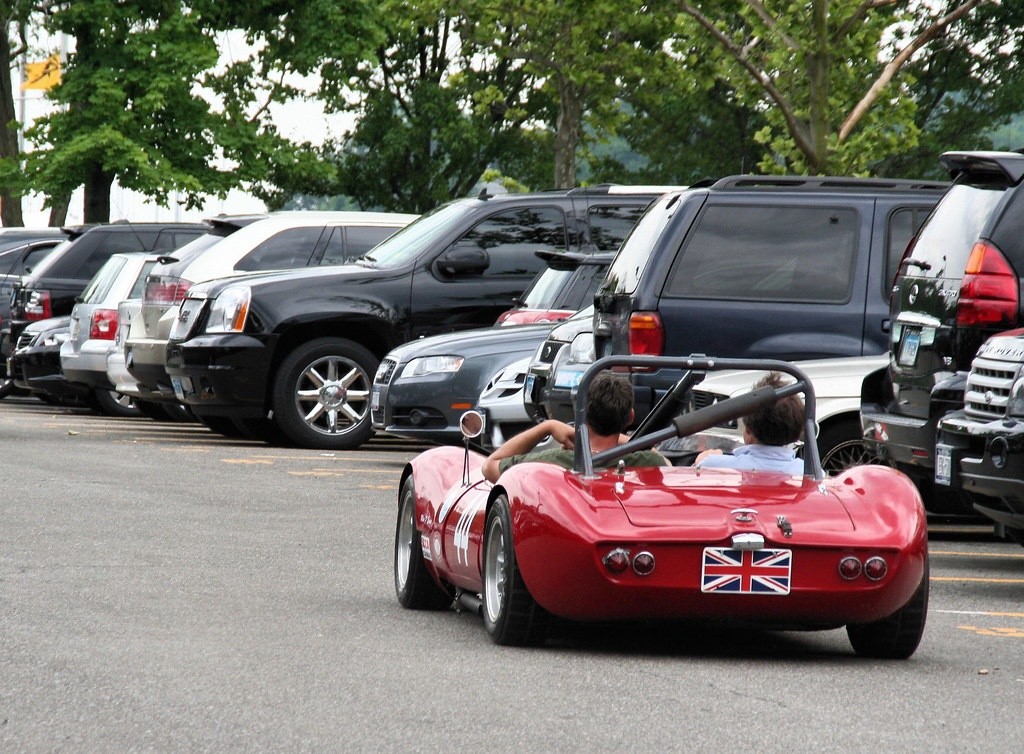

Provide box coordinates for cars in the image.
[6,314,100,407]
[368,248,635,447]
[389,353,932,664]
[60,248,176,416]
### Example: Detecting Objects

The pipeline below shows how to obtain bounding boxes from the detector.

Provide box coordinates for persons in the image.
[695,372,827,478]
[481,372,672,483]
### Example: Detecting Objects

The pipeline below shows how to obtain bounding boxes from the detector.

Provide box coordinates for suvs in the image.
[160,184,690,463]
[856,148,1024,515]
[118,209,421,422]
[0,226,81,378]
[586,176,958,485]
[936,329,1024,538]
[7,221,213,352]
